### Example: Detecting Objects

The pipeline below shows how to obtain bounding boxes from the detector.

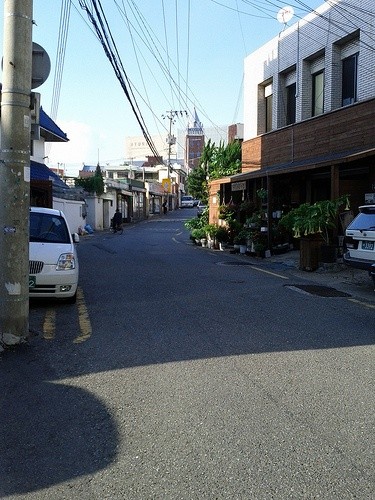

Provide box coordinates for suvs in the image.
[341,204,375,282]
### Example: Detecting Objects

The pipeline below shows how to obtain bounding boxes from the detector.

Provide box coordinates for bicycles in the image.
[109,218,123,235]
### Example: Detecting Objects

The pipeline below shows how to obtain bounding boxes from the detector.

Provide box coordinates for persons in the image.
[162,200,169,216]
[111,209,122,233]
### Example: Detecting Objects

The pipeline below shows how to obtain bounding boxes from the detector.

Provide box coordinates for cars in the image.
[181,195,194,208]
[196,200,208,217]
[29,206,80,304]
